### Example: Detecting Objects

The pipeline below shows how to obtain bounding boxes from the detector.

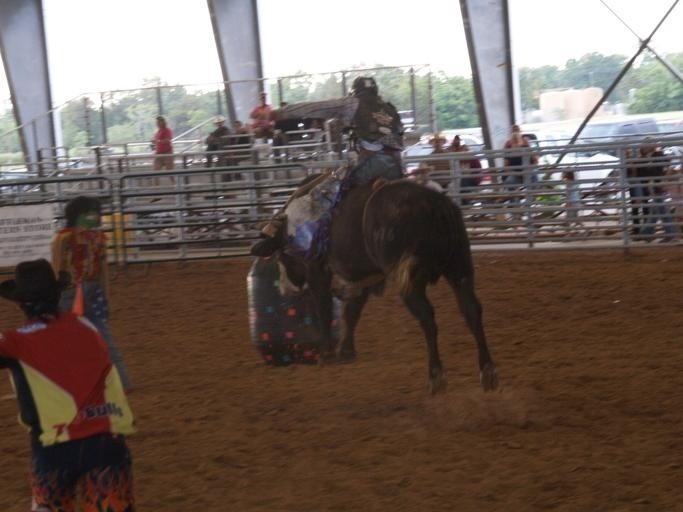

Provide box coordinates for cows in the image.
[250,173,500,395]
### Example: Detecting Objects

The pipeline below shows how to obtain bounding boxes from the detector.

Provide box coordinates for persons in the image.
[249,74,406,260]
[0,258,138,512]
[500,135,540,195]
[503,126,523,170]
[636,136,676,237]
[562,171,580,224]
[150,115,177,199]
[201,89,320,183]
[48,197,132,393]
[620,145,647,233]
[412,129,480,193]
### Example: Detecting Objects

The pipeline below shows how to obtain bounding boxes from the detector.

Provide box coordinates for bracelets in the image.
[157,140,160,144]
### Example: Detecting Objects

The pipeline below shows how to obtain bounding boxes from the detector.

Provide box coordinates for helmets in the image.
[347,76,378,96]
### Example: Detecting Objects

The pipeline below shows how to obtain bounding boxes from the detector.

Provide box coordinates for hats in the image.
[53,196,101,220]
[213,115,226,124]
[0,258,71,301]
[428,134,446,145]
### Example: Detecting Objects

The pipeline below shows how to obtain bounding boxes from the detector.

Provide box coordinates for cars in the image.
[398,109,682,193]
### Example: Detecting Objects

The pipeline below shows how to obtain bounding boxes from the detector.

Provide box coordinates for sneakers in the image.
[251,234,287,258]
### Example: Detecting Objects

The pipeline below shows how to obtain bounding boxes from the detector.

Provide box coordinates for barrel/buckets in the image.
[102,213,137,253]
[246,251,343,365]
